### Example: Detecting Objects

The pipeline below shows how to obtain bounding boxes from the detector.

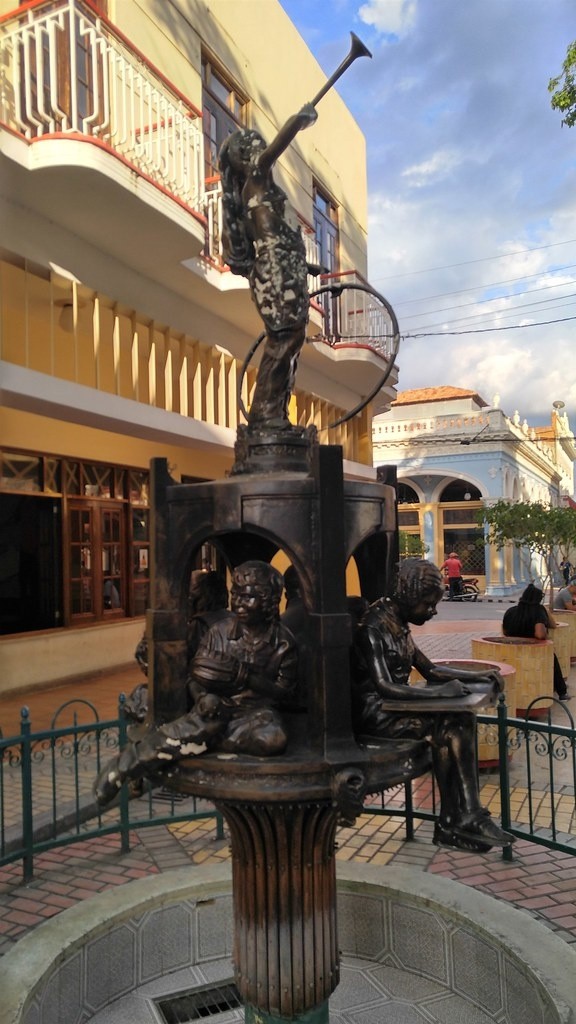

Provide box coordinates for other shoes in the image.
[446,598,466,602]
[559,693,571,702]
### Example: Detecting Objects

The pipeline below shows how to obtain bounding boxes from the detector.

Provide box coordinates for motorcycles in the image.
[441,574,479,602]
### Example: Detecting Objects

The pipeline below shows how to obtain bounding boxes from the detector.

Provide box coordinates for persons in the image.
[87,560,307,803]
[345,558,519,854]
[501,556,576,704]
[219,101,332,432]
[436,551,464,600]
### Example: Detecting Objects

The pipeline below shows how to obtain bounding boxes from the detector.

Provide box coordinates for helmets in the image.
[449,552,459,558]
